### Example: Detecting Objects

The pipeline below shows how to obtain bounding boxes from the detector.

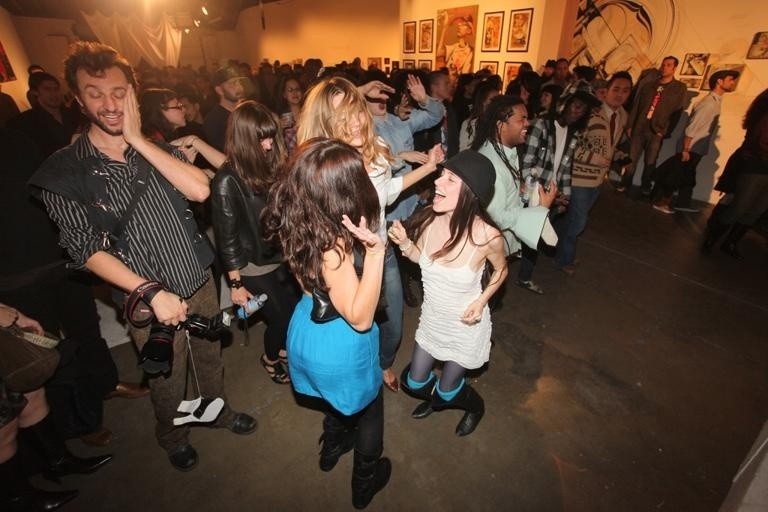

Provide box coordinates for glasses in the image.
[167,101,186,111]
[285,87,301,92]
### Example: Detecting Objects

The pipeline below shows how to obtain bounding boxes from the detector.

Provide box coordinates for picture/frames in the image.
[402,18,434,72]
[367,57,399,75]
[479,7,534,96]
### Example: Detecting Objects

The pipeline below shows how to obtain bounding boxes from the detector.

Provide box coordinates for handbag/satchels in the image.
[310,264,389,323]
[0,325,60,395]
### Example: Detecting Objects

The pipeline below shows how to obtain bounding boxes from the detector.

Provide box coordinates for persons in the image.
[382,146,509,437]
[23,40,258,472]
[2,268,150,451]
[300,55,603,393]
[615,55,688,196]
[555,73,634,276]
[702,91,768,261]
[0,300,114,510]
[652,70,736,215]
[260,137,393,512]
[2,54,324,382]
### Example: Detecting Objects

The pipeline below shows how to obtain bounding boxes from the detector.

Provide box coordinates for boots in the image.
[719,221,752,261]
[318,416,354,471]
[351,447,392,510]
[429,381,485,437]
[703,217,731,248]
[400,362,437,419]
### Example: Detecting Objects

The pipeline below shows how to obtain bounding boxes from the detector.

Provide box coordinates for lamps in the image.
[182,0,211,35]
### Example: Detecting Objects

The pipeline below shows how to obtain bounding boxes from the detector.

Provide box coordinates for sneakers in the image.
[514,279,543,295]
[226,413,258,435]
[615,184,701,214]
[168,443,198,472]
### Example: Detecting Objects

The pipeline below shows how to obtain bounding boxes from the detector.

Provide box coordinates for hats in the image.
[210,66,248,87]
[435,149,497,214]
[564,79,601,108]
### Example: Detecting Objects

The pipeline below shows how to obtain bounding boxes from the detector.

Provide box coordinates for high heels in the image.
[260,353,290,383]
[278,349,287,368]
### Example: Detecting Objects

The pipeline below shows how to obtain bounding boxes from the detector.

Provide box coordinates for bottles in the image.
[236,292,270,322]
[522,169,541,209]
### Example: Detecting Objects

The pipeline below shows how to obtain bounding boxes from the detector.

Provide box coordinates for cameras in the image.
[138,312,231,375]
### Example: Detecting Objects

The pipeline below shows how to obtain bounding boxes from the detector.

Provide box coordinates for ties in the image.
[609,112,617,146]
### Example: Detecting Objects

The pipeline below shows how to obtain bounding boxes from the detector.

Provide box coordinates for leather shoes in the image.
[1,381,152,512]
[382,368,399,392]
